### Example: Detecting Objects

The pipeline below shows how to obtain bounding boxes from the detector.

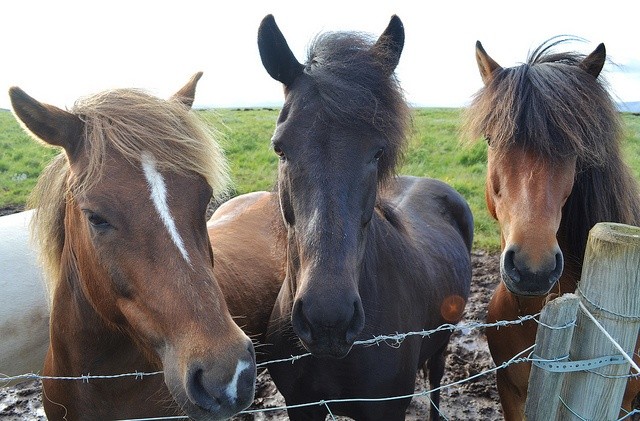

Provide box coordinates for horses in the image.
[8,70,287,421]
[455,34,639,421]
[257,13,475,420]
[0,202,52,388]
